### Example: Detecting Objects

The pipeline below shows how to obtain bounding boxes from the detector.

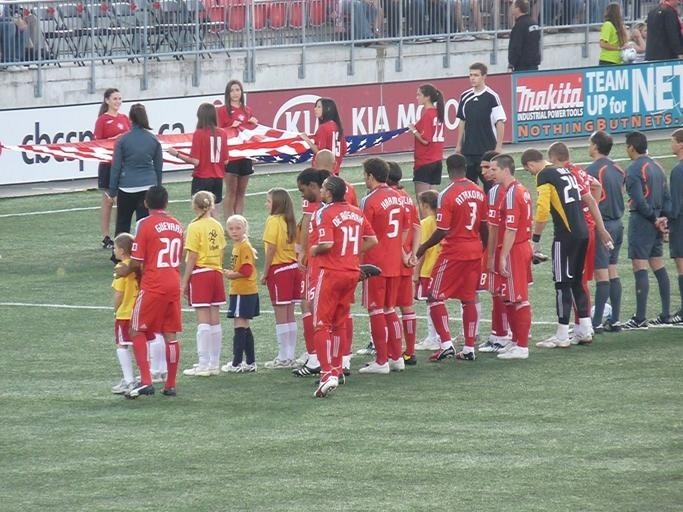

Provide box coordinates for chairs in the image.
[42,0,333,67]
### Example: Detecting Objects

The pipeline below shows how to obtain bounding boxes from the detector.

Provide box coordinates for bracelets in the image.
[531,233,540,244]
[412,130,417,135]
[174,151,180,158]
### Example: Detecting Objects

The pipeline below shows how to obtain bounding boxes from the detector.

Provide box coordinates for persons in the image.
[507,1,541,71]
[326,1,515,45]
[533,0,611,34]
[663,128,682,329]
[110,184,184,400]
[618,130,674,331]
[102,103,164,266]
[88,87,132,253]
[599,3,627,65]
[406,83,448,202]
[579,129,626,334]
[212,77,260,229]
[260,149,534,399]
[184,192,260,377]
[164,102,229,226]
[518,149,594,352]
[545,141,615,341]
[624,1,683,61]
[455,62,508,194]
[297,95,348,179]
[0,0,54,64]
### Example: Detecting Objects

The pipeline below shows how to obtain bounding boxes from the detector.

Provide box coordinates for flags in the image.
[0,113,411,170]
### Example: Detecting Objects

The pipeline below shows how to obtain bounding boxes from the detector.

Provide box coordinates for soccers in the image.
[590,304,611,326]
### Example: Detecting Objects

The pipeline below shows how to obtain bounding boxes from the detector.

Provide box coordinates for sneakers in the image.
[181,361,256,376]
[413,328,532,362]
[356,339,417,374]
[313,374,346,399]
[110,372,176,398]
[535,316,621,349]
[98,234,114,249]
[621,307,683,330]
[263,356,349,376]
